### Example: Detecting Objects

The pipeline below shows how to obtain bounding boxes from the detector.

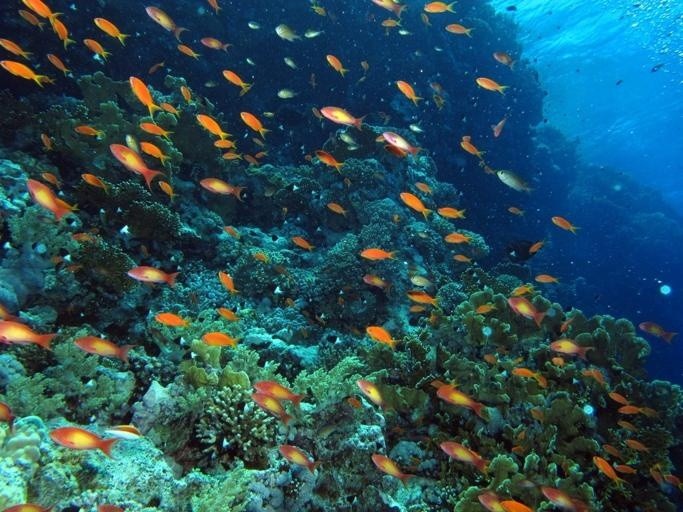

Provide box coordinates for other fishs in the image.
[0,1,683,512]
